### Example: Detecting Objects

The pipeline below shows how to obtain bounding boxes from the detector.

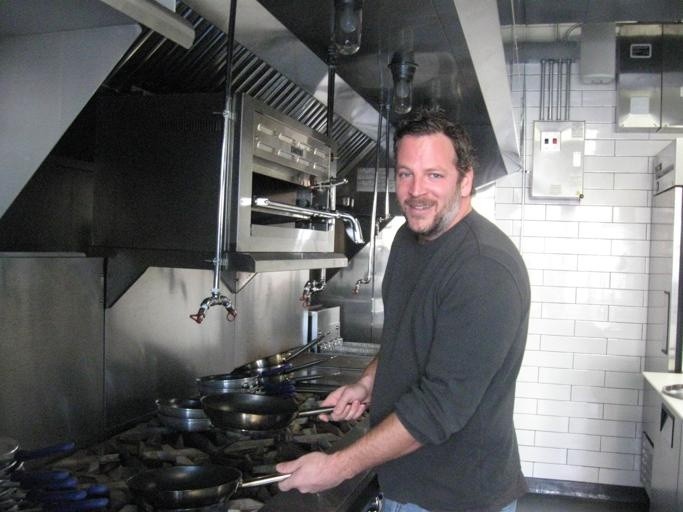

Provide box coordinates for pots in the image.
[0,431,110,511]
[126,463,293,510]
[149,331,368,442]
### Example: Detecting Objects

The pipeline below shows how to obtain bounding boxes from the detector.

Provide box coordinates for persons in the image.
[275,111,530,511]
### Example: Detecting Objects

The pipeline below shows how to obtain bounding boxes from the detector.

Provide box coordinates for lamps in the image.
[333,0,418,115]
